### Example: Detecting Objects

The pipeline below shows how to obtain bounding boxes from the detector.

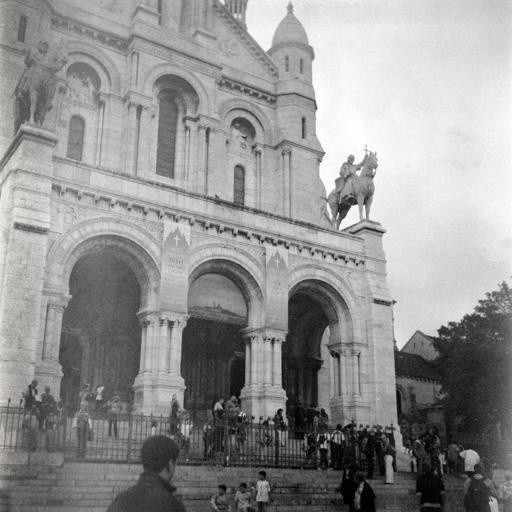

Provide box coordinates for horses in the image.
[327,151,378,230]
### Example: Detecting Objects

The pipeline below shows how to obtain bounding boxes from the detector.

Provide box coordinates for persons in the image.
[457,448,496,511]
[18,375,119,459]
[234,482,255,511]
[106,435,189,511]
[340,152,367,197]
[210,484,233,511]
[402,415,478,478]
[254,471,274,511]
[157,392,394,485]
[415,462,444,512]
[483,451,511,511]
[340,454,358,509]
[352,471,376,512]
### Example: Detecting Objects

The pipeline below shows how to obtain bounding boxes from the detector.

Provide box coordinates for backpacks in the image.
[465,474,489,511]
